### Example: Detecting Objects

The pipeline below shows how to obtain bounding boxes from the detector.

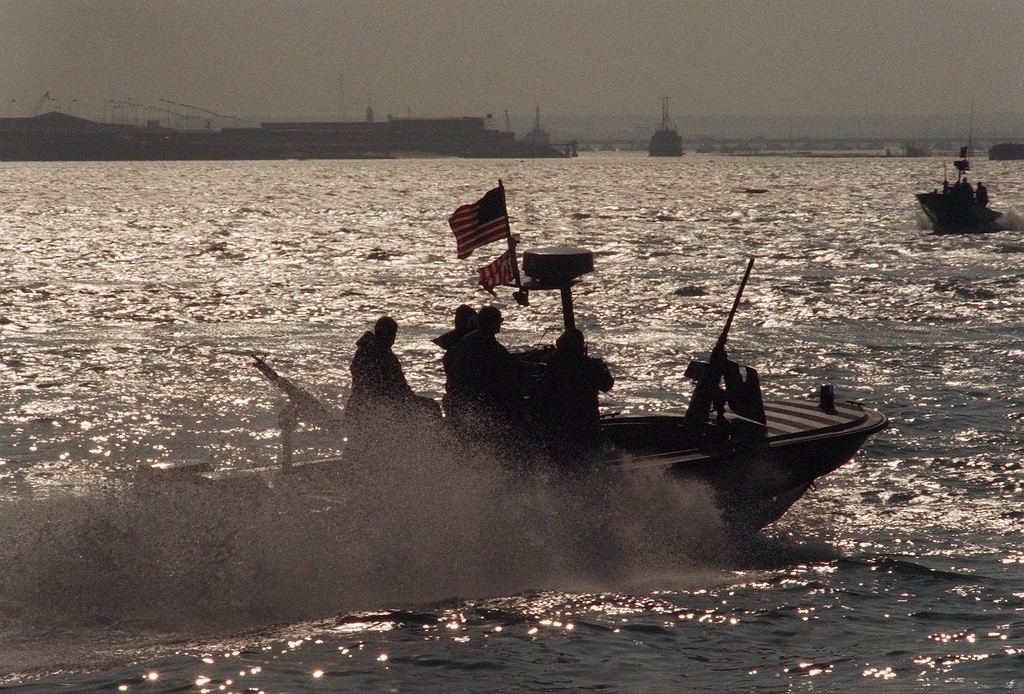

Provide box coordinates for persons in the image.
[342,317,444,432]
[431,304,479,350]
[527,325,616,423]
[447,302,523,395]
[962,178,974,196]
[977,180,989,207]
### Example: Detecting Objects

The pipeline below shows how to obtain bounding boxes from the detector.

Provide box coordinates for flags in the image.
[449,184,509,260]
[476,250,515,291]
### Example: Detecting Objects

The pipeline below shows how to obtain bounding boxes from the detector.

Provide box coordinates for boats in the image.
[646,96,684,155]
[111,243,886,581]
[915,161,1006,240]
